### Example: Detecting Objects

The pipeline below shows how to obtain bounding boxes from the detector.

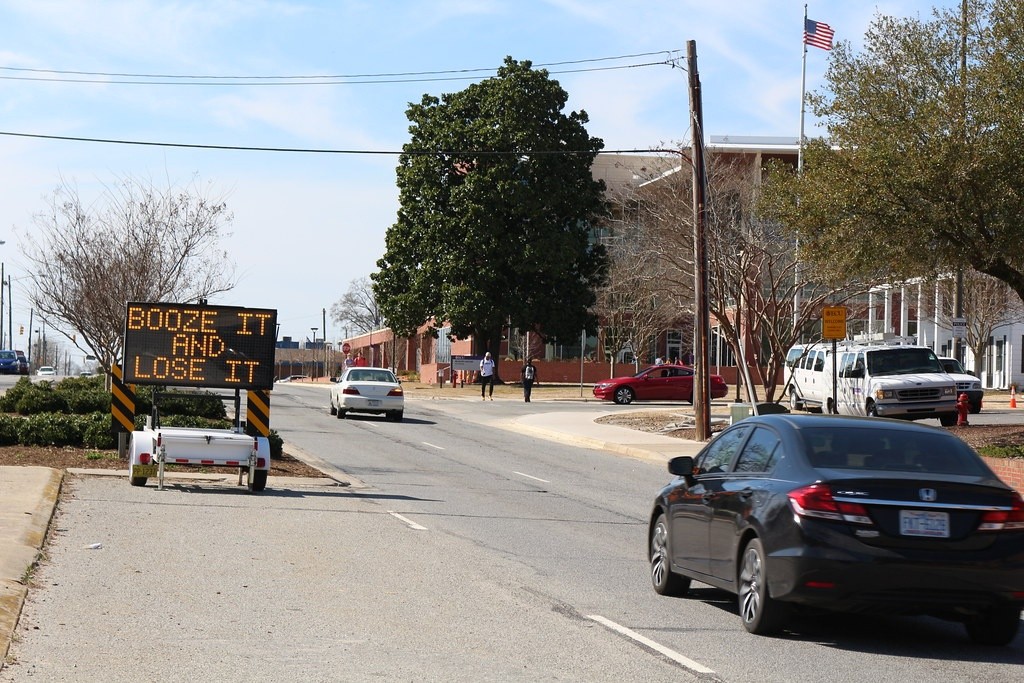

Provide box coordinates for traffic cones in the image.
[1009,385,1017,408]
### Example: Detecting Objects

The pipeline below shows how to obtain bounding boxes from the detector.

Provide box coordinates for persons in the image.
[661,370,668,377]
[345,352,368,369]
[656,356,683,366]
[520,359,539,402]
[480,352,495,401]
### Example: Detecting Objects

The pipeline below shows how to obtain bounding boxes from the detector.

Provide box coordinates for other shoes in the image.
[483,396,485,401]
[488,395,493,401]
[525,396,530,402]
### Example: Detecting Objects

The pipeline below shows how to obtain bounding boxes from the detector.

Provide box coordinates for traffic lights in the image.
[20,326,24,335]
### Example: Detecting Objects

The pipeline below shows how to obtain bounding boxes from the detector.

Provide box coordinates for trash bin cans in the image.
[729,402,787,425]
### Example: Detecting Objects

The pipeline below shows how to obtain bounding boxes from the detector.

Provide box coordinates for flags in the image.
[803,18,835,51]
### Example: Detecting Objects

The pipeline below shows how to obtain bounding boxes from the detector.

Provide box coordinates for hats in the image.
[527,358,532,363]
[486,352,491,357]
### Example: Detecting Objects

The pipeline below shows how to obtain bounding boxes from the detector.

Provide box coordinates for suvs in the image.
[822,345,957,427]
[936,356,984,414]
[16,351,31,374]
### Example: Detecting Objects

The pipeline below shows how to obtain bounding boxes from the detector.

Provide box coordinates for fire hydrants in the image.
[955,392,973,426]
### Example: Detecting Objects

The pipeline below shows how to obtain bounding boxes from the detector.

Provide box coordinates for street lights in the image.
[311,328,318,381]
[9,274,48,350]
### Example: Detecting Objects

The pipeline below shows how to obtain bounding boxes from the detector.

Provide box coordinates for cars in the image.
[329,367,403,421]
[276,375,308,383]
[593,365,729,405]
[36,366,57,375]
[649,413,1024,643]
[0,350,20,374]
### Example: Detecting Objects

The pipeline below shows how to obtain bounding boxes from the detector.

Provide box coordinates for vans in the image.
[80,372,91,377]
[784,341,845,411]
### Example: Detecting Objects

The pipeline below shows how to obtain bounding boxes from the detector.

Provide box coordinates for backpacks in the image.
[524,365,534,379]
